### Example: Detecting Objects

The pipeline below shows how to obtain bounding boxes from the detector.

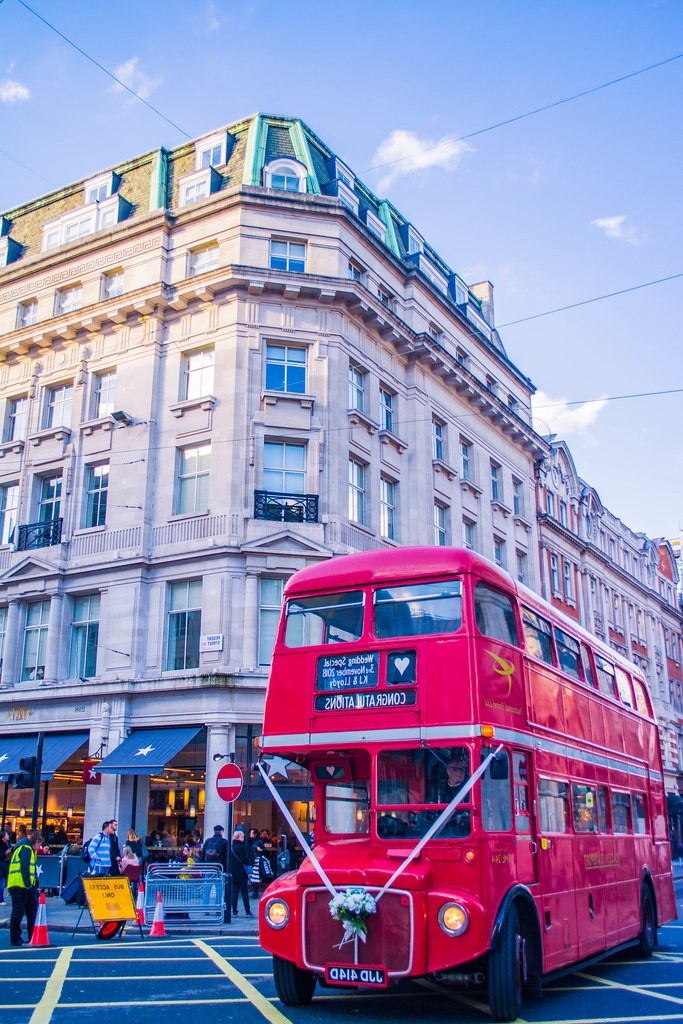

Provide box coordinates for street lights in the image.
[212,752,237,923]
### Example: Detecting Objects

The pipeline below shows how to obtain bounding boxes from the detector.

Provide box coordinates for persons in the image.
[0,822,69,854]
[574,808,598,831]
[426,757,471,819]
[201,825,229,917]
[7,831,45,947]
[125,828,144,906]
[118,846,140,905]
[226,831,256,917]
[0,830,13,905]
[88,820,122,877]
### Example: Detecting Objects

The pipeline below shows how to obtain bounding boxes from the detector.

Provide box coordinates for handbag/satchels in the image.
[243,864,253,875]
[259,856,274,880]
[139,838,149,862]
[175,845,190,867]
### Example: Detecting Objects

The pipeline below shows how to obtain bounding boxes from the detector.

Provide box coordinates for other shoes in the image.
[246,913,253,918]
[232,911,238,917]
[0,902,6,906]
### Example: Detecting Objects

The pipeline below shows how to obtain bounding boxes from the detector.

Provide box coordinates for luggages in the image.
[60,871,87,905]
[76,859,106,908]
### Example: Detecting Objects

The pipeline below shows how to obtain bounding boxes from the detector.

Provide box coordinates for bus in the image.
[251,546,679,1023]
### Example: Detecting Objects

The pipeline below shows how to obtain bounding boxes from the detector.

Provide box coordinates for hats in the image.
[214,825,224,831]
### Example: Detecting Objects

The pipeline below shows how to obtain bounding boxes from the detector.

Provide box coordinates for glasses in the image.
[448,765,465,773]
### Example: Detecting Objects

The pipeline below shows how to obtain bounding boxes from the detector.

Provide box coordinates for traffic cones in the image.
[29,893,52,947]
[147,891,170,939]
[132,882,148,927]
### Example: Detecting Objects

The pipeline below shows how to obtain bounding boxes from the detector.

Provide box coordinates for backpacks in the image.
[81,833,103,863]
[205,837,221,865]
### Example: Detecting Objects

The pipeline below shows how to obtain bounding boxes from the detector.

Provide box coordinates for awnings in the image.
[146,822,314,898]
[92,728,201,776]
[0,735,89,782]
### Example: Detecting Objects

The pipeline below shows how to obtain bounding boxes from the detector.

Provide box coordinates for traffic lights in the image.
[8,756,36,789]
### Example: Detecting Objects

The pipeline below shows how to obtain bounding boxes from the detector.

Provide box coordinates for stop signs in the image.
[217,763,243,804]
